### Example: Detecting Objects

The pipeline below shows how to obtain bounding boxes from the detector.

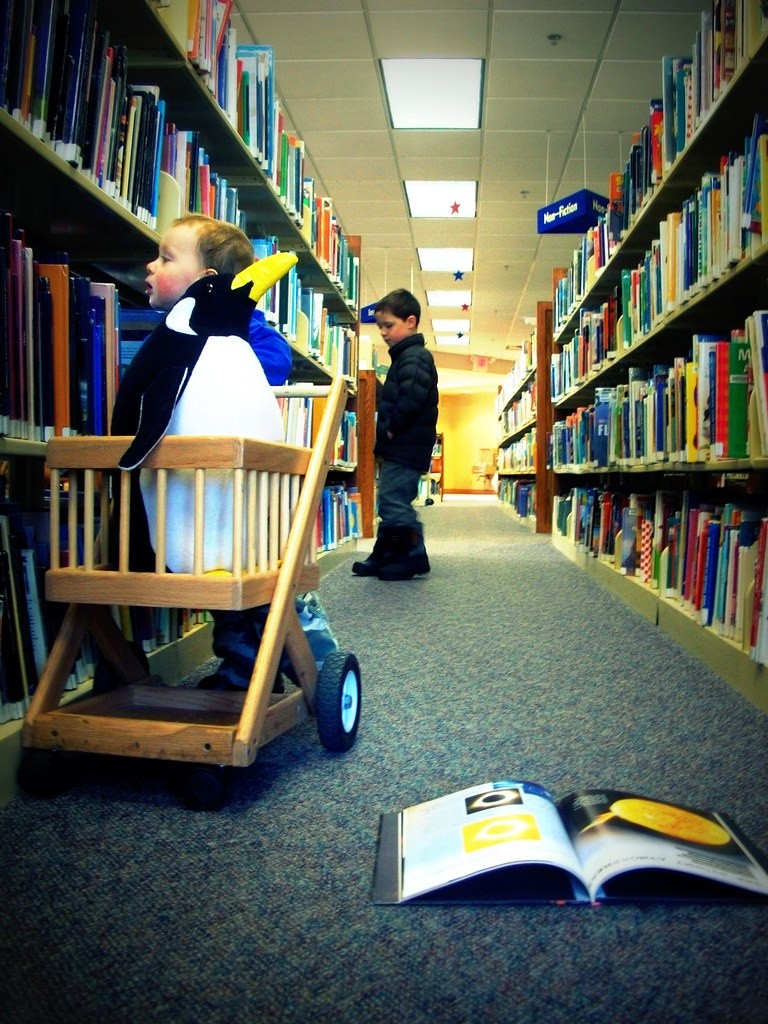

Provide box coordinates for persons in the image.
[351,288,439,581]
[145,212,339,693]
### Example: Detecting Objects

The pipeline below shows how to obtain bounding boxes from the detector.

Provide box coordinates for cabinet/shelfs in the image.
[496,0,768,715]
[0,0,391,805]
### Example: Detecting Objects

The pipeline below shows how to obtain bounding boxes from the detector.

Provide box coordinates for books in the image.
[551,0,768,664]
[495,328,536,517]
[368,779,768,907]
[1,0,360,726]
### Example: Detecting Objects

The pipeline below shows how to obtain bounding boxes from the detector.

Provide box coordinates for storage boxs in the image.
[537,189,610,235]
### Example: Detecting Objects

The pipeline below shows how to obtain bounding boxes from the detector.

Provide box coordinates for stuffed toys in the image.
[110,253,299,579]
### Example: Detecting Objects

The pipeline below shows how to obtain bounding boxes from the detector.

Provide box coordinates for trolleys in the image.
[18,376,364,809]
